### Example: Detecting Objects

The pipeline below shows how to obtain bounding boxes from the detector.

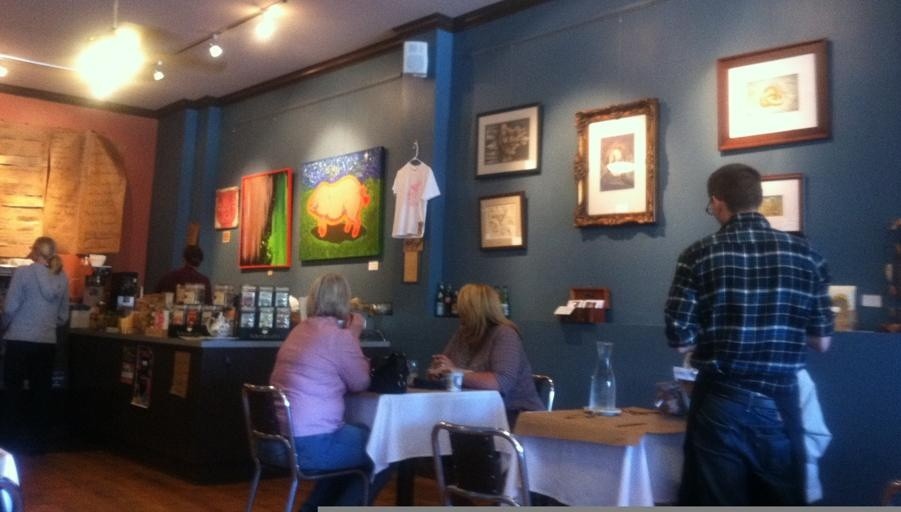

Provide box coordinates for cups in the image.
[444,374,464,392]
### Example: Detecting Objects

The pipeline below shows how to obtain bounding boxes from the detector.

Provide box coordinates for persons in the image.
[170,245,214,304]
[1,236,72,453]
[664,162,837,506]
[269,273,402,512]
[687,348,834,503]
[427,278,546,483]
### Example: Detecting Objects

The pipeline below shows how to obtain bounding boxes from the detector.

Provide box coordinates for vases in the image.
[589,341,618,413]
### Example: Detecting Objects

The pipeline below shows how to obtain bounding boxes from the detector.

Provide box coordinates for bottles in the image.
[586,339,622,413]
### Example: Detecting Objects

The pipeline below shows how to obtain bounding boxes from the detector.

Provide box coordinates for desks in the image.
[352,388,504,501]
[517,407,709,507]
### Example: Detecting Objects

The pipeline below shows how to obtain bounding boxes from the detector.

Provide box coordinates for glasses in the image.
[705,201,712,216]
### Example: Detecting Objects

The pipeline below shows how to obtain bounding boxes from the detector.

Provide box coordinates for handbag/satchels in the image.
[368,351,408,393]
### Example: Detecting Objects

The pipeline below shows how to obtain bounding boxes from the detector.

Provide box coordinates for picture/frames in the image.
[236,167,293,268]
[474,101,545,181]
[479,189,529,252]
[574,94,661,228]
[715,36,831,152]
[759,174,805,234]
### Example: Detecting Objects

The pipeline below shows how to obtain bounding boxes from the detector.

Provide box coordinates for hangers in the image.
[408,141,425,166]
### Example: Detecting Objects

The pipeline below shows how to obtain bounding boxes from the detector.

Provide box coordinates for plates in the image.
[582,406,623,417]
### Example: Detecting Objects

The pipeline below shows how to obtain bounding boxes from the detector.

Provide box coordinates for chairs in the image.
[235,383,370,509]
[529,370,558,414]
[427,421,528,505]
